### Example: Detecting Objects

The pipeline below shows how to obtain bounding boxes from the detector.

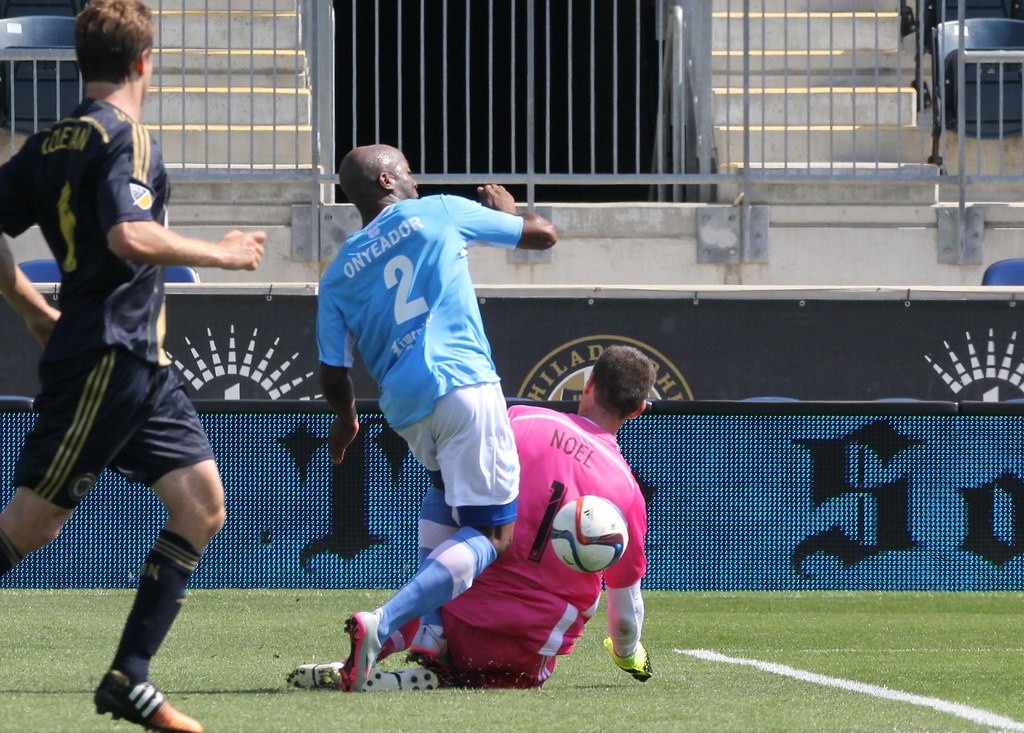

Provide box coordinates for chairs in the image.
[931,17,1024,168]
[916,0,1023,105]
[0,14,89,134]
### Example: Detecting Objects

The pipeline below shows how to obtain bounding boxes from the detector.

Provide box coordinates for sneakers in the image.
[282,610,452,693]
[93,672,206,733]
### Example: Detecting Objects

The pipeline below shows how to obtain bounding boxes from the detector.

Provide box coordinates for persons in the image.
[0,0,270,733]
[288,345,657,693]
[314,143,558,695]
